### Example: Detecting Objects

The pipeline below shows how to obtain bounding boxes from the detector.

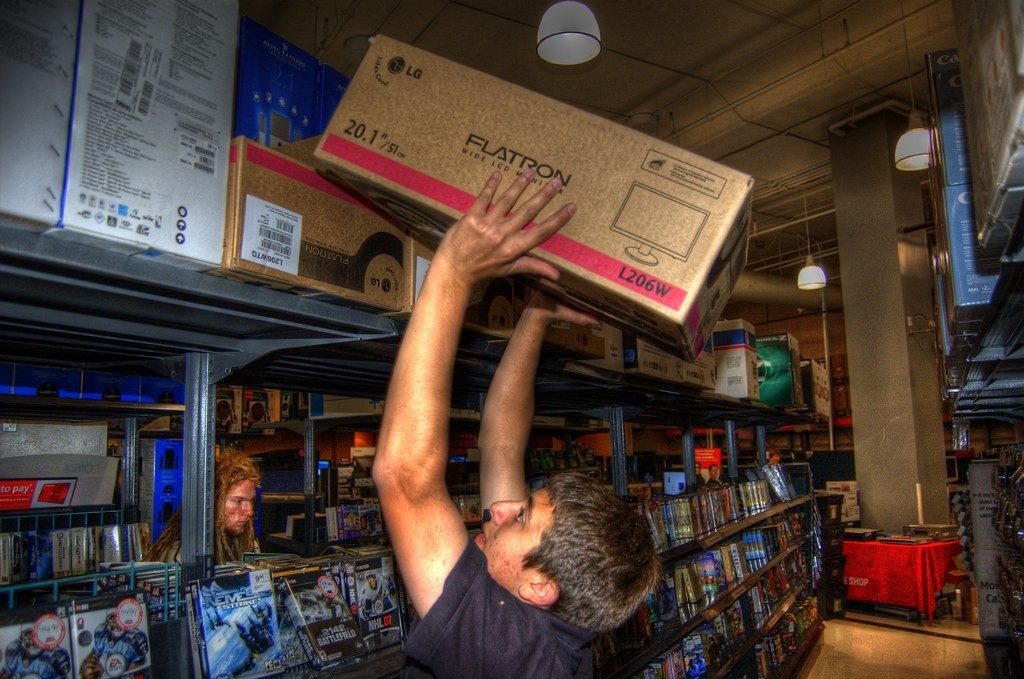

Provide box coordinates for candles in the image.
[916,482,924,524]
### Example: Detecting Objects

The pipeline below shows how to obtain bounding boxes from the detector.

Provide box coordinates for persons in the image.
[706,463,722,486]
[695,462,706,486]
[371,167,659,679]
[707,637,721,665]
[768,447,781,463]
[142,445,262,564]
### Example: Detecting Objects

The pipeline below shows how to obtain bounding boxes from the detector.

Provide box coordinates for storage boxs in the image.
[41,0,238,272]
[751,333,804,409]
[473,281,604,360]
[313,35,750,364]
[622,331,678,384]
[576,321,622,374]
[712,319,759,398]
[0,0,83,233]
[201,136,403,313]
[793,358,828,418]
[814,480,860,623]
[678,333,716,390]
[381,234,515,345]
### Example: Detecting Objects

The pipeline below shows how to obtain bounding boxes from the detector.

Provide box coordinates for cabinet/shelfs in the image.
[0,225,826,679]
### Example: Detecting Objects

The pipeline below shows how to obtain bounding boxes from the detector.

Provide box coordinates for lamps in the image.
[894,0,931,171]
[536,0,601,66]
[796,186,826,290]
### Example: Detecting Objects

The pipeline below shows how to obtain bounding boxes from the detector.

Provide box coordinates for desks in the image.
[844,533,965,627]
